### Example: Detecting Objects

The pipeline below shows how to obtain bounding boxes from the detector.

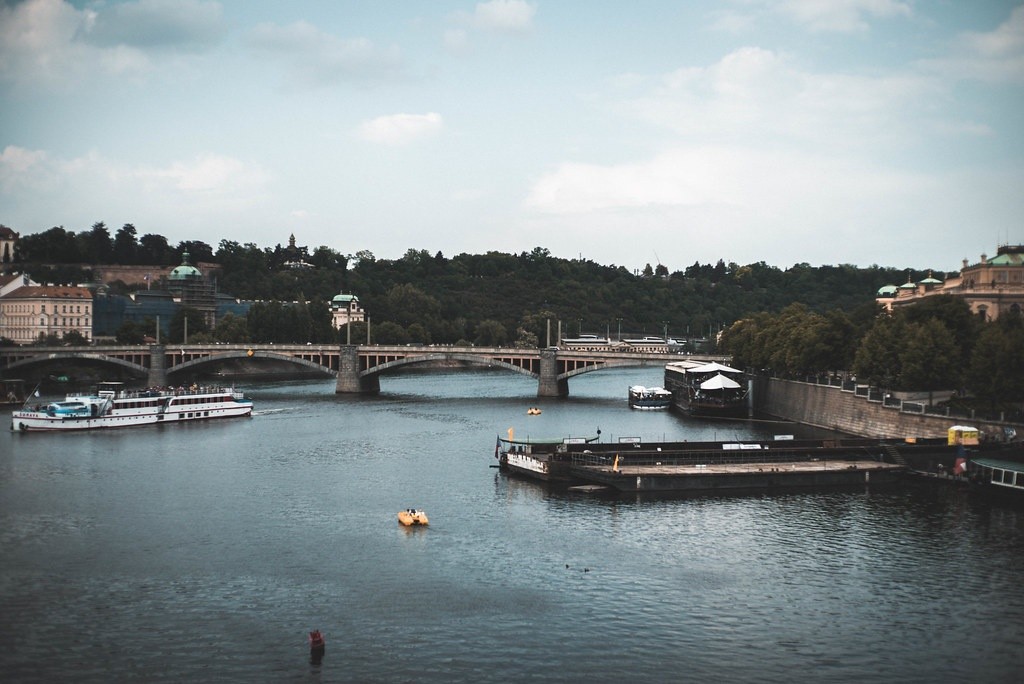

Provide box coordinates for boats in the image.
[9,382,256,433]
[396,508,431,527]
[663,358,744,421]
[627,384,672,412]
[527,408,543,416]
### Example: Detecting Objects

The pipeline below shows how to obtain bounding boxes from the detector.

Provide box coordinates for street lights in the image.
[616,316,624,341]
[662,320,671,344]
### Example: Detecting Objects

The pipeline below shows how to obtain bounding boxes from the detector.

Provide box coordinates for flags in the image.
[508,428,513,442]
[495,432,501,458]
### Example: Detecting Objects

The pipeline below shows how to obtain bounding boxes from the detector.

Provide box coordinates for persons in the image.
[405,507,424,518]
[134,381,226,398]
